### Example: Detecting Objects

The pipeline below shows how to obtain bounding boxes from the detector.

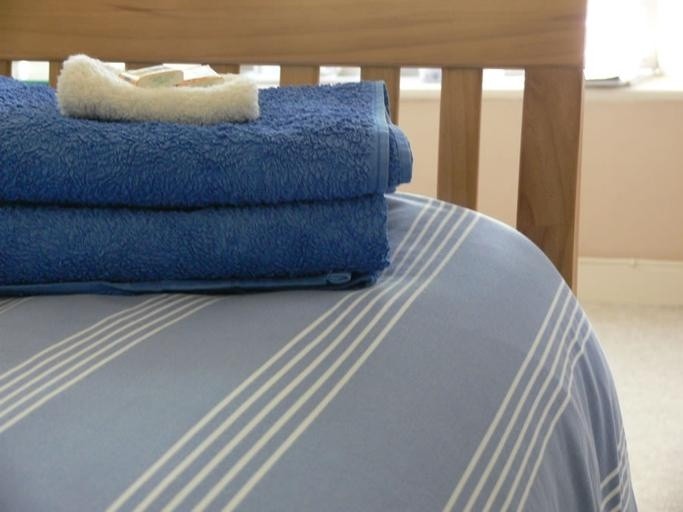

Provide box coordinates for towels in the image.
[0,74,413,209]
[1,193,391,295]
[56,53,261,125]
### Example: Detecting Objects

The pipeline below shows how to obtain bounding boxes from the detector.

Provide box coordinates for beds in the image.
[0,0,637,512]
